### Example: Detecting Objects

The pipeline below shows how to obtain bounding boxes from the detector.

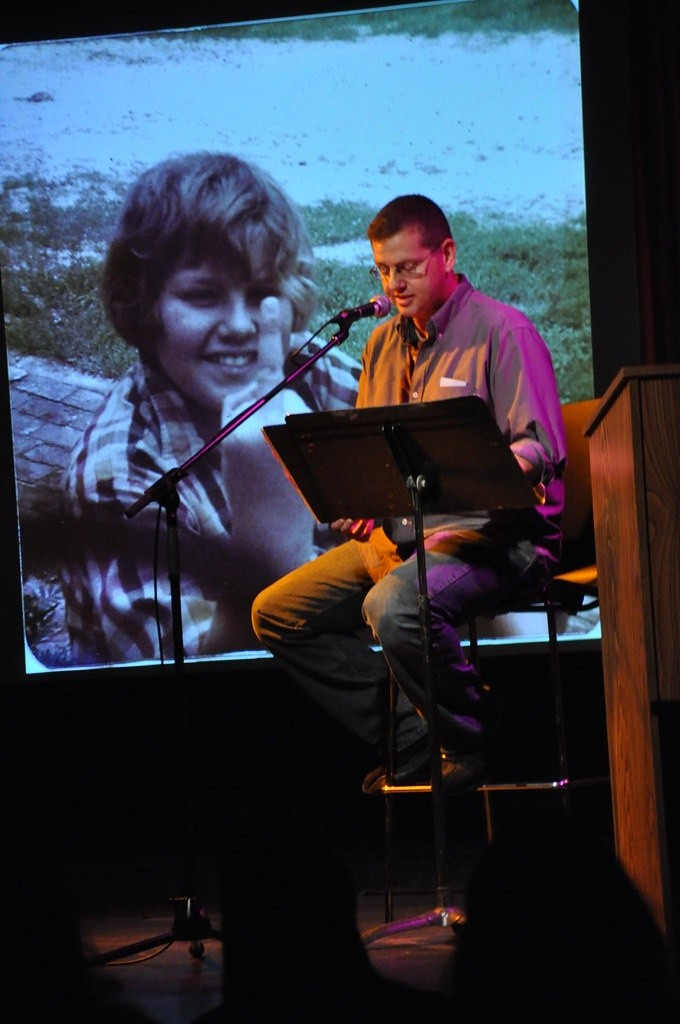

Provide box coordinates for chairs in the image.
[379,397,609,936]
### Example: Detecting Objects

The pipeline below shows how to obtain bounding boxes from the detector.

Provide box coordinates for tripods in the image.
[263,399,544,947]
[97,328,352,965]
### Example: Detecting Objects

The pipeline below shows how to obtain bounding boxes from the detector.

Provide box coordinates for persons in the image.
[250,196,567,796]
[0,841,164,1024]
[65,155,363,665]
[188,802,452,1024]
[443,798,680,1024]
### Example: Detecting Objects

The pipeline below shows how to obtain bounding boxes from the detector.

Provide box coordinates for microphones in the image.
[329,295,392,323]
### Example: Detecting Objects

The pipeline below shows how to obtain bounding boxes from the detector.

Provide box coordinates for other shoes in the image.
[363,733,432,793]
[430,737,490,792]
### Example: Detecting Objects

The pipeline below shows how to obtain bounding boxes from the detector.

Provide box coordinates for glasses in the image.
[369,245,441,283]
